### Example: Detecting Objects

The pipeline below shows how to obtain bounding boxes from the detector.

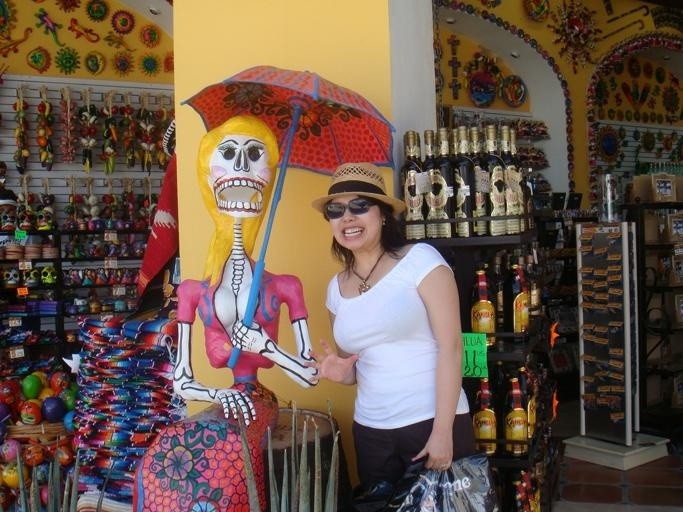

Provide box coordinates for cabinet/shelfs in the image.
[0,225,156,379]
[402,201,682,512]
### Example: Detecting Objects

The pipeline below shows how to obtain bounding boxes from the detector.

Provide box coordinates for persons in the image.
[311,161,497,511]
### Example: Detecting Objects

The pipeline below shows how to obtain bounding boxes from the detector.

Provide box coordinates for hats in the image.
[311,163,406,216]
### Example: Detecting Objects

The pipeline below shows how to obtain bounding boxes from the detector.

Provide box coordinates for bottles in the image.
[638,160,683,176]
[471,361,554,511]
[468,241,540,334]
[399,124,536,236]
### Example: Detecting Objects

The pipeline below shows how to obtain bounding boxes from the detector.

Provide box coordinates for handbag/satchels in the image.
[352,452,499,512]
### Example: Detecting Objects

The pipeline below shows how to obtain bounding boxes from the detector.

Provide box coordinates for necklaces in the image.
[350,252,386,296]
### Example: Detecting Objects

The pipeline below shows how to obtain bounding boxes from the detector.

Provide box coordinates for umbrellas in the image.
[178,66,399,367]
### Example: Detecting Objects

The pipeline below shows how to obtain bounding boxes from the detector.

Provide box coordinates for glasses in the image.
[325,198,377,218]
